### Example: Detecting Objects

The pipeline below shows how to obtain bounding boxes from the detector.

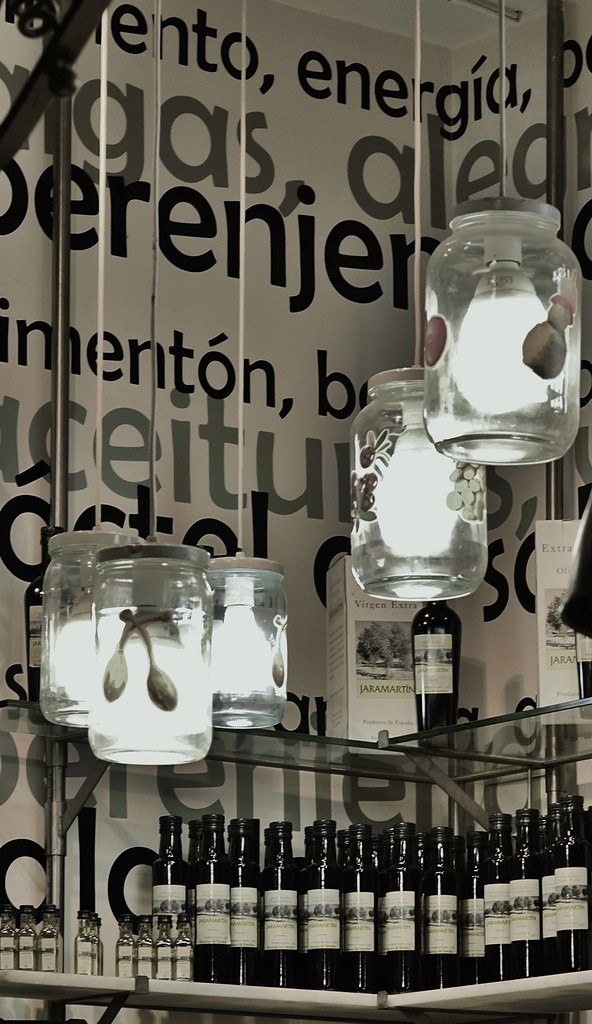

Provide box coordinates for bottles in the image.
[574,632,592,720]
[38,531,291,767]
[74,910,104,976]
[152,794,592,993]
[0,904,63,973]
[348,364,490,602]
[422,196,582,465]
[115,913,194,982]
[411,600,463,746]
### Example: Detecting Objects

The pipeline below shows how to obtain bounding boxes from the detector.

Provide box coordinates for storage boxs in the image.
[326,555,426,754]
[535,519,592,725]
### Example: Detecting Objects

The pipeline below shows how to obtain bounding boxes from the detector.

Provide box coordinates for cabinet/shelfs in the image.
[0,697,592,1024]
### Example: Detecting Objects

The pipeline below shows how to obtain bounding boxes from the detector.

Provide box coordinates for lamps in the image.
[43,0,585,762]
